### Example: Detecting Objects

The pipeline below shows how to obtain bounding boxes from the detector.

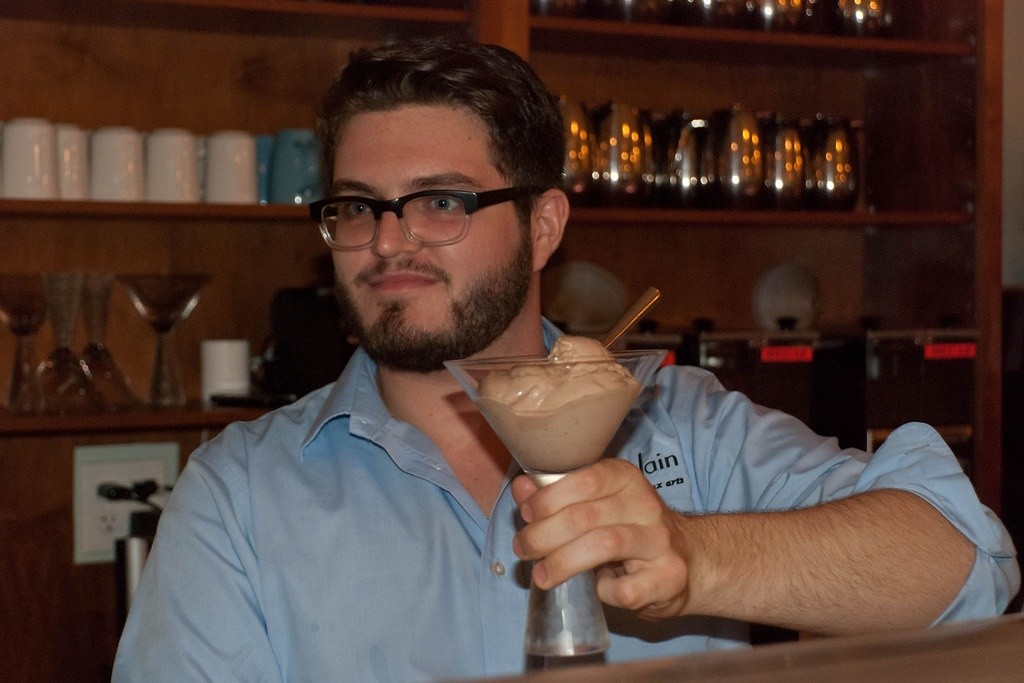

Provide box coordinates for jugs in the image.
[714,106,769,211]
[539,0,886,37]
[595,103,657,207]
[809,117,860,212]
[766,115,808,208]
[553,96,596,205]
[658,109,719,209]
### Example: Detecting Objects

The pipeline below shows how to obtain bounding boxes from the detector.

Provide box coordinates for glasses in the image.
[310,185,545,251]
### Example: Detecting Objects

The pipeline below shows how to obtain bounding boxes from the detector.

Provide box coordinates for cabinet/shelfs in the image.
[0,0,1003,683]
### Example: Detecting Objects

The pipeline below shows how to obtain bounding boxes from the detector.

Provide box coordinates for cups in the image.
[146,129,201,204]
[257,136,274,205]
[196,131,259,205]
[90,125,146,203]
[200,340,250,407]
[1,118,89,200]
[271,128,327,204]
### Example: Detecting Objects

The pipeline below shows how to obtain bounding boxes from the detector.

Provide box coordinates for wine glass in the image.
[114,271,215,412]
[443,349,669,658]
[77,273,142,412]
[0,270,51,414]
[33,269,93,414]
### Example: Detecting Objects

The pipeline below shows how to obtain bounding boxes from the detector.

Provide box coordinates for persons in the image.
[109,36,1020,683]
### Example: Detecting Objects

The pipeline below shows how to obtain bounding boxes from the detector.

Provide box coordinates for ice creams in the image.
[476,338,644,475]
